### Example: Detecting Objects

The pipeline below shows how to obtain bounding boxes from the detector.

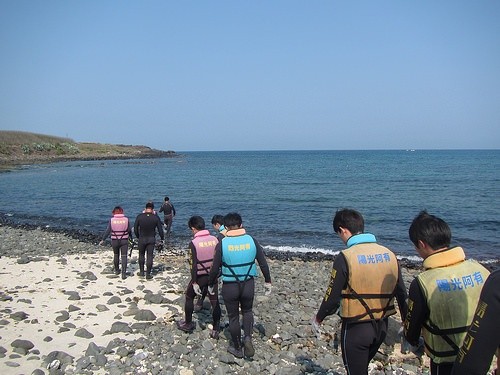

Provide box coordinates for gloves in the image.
[99,240,104,246]
[161,240,165,245]
[208,286,215,295]
[411,338,425,356]
[193,284,203,296]
[264,283,271,295]
[401,339,410,354]
[312,320,321,337]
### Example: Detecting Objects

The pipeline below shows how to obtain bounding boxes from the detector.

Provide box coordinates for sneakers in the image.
[212,330,219,339]
[242,335,255,357]
[176,320,194,332]
[227,345,243,358]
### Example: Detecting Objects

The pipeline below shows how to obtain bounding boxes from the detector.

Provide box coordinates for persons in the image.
[451,269,500,375]
[208,212,271,359]
[159,197,176,237]
[134,203,166,279]
[404,209,491,375]
[99,206,135,280]
[194,214,228,312]
[176,216,221,340]
[311,208,409,375]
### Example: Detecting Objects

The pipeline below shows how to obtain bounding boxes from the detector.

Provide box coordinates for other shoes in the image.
[194,305,202,311]
[137,272,145,276]
[146,275,153,279]
